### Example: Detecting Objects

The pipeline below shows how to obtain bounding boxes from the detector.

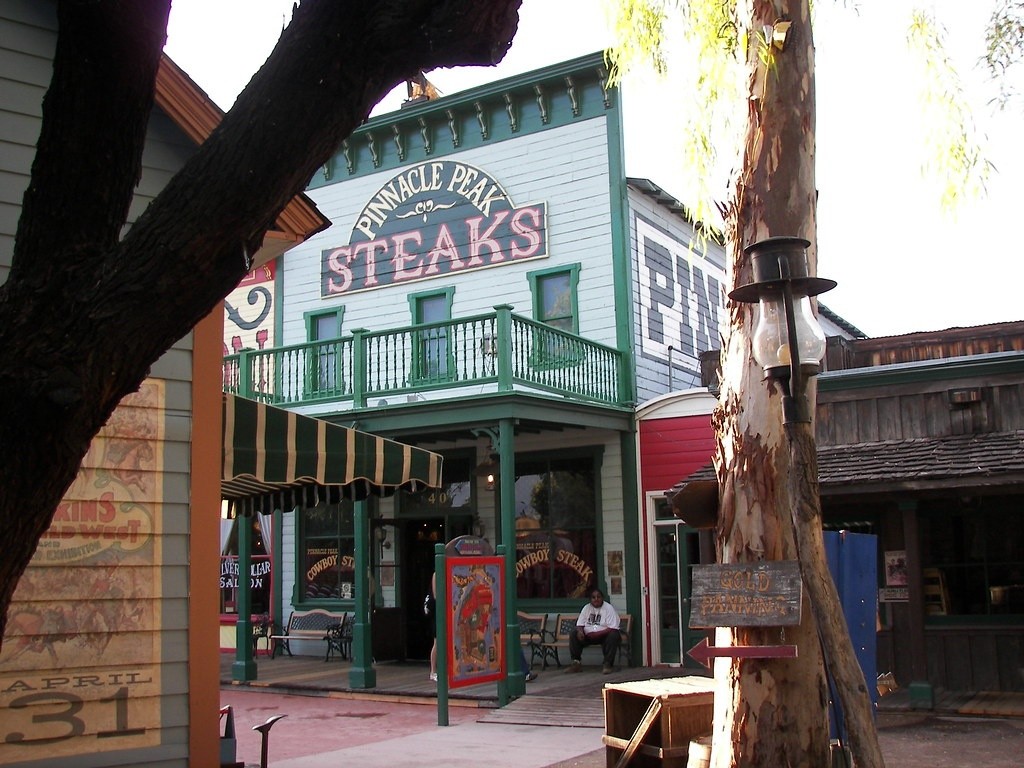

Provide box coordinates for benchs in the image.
[517,610,550,671]
[539,614,634,672]
[263,609,354,662]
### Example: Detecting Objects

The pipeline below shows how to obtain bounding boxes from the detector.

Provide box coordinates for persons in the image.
[429,571,437,682]
[521,645,537,682]
[569,589,620,673]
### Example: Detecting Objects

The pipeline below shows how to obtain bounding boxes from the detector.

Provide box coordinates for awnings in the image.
[223,392,442,519]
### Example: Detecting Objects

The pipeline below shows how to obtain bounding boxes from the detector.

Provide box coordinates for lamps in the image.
[472,435,498,491]
[375,526,390,550]
[471,511,489,544]
[727,235,837,427]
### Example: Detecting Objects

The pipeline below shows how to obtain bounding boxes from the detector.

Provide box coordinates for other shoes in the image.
[525,671,538,682]
[430,672,438,681]
[564,661,583,673]
[603,665,611,674]
